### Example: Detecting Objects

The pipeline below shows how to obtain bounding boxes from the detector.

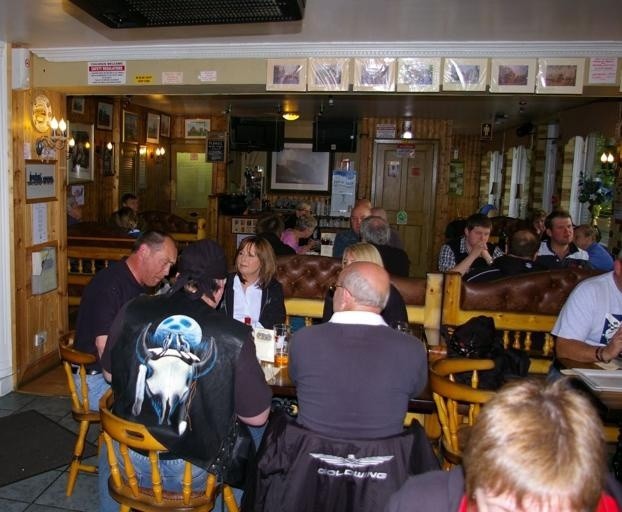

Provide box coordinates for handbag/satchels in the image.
[442,315,509,390]
[406,418,443,474]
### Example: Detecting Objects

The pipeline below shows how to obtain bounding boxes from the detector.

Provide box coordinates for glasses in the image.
[329,285,352,296]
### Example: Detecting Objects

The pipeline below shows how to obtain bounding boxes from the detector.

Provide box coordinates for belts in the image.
[70,367,102,376]
[127,444,178,461]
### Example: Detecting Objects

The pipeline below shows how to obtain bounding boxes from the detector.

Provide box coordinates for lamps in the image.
[601,149,615,169]
[281,111,302,120]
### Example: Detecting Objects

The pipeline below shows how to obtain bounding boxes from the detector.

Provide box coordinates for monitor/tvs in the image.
[312,120,357,153]
[228,116,285,152]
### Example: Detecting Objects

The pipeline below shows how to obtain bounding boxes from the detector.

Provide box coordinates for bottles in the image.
[310,196,324,211]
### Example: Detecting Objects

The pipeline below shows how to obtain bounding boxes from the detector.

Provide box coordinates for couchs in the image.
[67,221,206,318]
[444,216,536,251]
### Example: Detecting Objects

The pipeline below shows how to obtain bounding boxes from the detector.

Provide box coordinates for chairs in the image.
[98,388,241,512]
[58,331,100,496]
[428,347,622,472]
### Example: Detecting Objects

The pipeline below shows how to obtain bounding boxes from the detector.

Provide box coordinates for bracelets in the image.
[595,346,604,362]
[600,347,612,364]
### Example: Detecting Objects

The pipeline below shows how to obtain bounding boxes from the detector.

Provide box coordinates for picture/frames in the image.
[25,159,60,203]
[67,96,211,185]
[266,137,334,196]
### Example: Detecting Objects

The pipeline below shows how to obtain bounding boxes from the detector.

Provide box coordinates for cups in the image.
[319,219,350,228]
[274,324,292,364]
[390,320,412,335]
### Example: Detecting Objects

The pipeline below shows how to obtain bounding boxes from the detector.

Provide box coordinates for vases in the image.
[589,204,614,231]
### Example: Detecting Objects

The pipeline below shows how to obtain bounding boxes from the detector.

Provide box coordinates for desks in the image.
[262,360,436,415]
[556,357,622,427]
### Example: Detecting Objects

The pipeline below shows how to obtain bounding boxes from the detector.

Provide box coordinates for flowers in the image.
[579,170,612,205]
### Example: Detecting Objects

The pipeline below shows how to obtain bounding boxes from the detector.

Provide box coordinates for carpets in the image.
[1,410,99,486]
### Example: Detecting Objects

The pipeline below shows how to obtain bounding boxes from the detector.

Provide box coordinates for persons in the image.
[93,206,147,240]
[215,236,285,336]
[68,225,178,412]
[98,239,272,512]
[288,261,441,512]
[384,370,622,512]
[437,209,613,282]
[321,241,409,331]
[264,201,409,277]
[549,249,622,388]
[121,193,152,233]
